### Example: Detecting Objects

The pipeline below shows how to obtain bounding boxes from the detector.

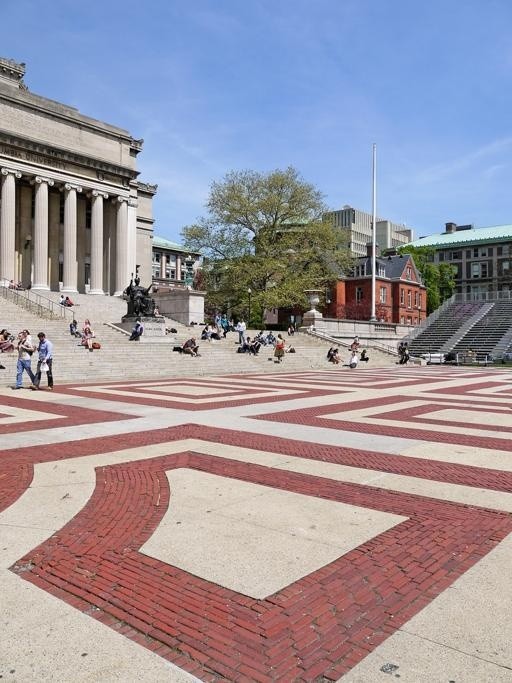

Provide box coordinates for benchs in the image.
[407,301,512,366]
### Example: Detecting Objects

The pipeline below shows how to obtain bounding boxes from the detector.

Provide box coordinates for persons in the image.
[65,296,73,307]
[9,279,15,290]
[326,336,369,369]
[183,337,199,357]
[126,277,154,316]
[0,329,14,353]
[30,332,54,392]
[23,330,33,357]
[59,295,65,306]
[201,314,295,363]
[81,327,94,351]
[129,320,143,341]
[82,318,95,338]
[13,332,35,390]
[404,342,410,364]
[16,280,25,290]
[399,342,405,363]
[70,320,82,338]
[472,350,477,356]
[154,305,159,315]
[467,349,472,356]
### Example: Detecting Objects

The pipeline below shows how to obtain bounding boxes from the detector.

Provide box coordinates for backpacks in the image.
[18,339,33,355]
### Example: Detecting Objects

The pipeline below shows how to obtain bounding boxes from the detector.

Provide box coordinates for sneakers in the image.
[30,384,38,389]
